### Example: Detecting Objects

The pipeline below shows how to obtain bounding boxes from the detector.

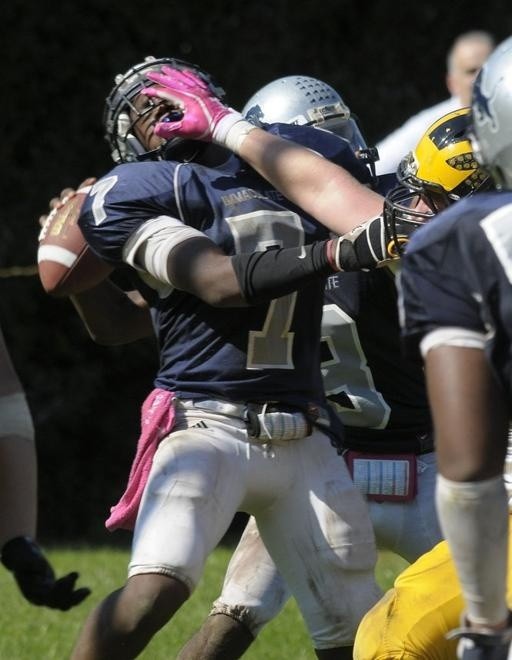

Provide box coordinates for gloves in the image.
[445,610,512,660]
[0,536,91,611]
[336,210,421,273]
[140,66,245,148]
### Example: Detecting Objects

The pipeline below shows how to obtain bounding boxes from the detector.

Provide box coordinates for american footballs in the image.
[36,184,114,296]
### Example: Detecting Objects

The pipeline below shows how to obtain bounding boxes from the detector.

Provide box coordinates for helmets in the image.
[105,56,227,164]
[242,75,379,189]
[384,107,497,258]
[467,36,512,192]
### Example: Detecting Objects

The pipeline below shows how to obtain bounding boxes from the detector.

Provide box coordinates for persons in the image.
[1,28,510,660]
